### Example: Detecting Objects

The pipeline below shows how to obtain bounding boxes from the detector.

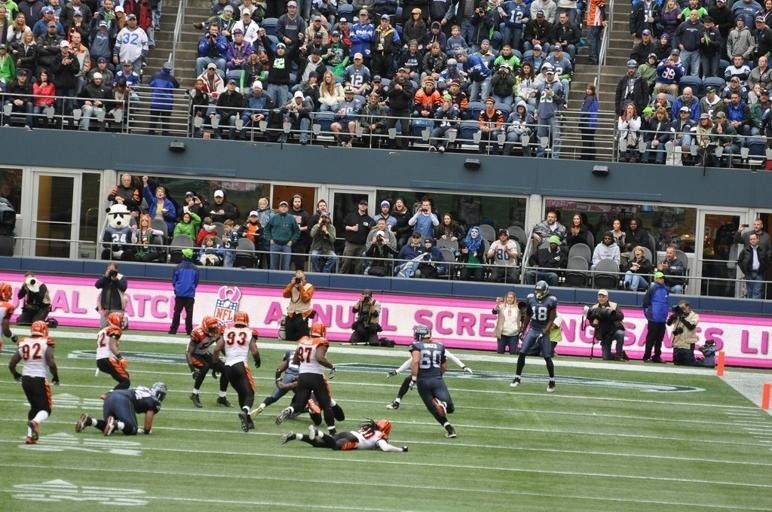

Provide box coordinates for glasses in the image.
[604,237,611,241]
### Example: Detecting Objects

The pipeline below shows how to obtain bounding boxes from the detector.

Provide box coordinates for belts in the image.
[751,268,759,274]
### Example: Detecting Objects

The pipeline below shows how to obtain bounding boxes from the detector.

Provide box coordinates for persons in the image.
[94,261,129,330]
[76,381,168,437]
[98,172,766,300]
[509,279,559,393]
[666,300,704,368]
[0,0,178,138]
[185,315,228,408]
[537,310,562,359]
[282,270,314,340]
[211,310,261,430]
[305,371,345,426]
[347,288,395,348]
[614,1,772,172]
[0,282,19,354]
[250,347,302,420]
[95,309,130,400]
[586,288,625,362]
[15,270,59,329]
[492,291,522,354]
[384,342,474,410]
[167,249,198,335]
[186,2,606,160]
[8,320,60,445]
[642,271,670,363]
[280,416,409,453]
[409,323,457,439]
[275,321,338,437]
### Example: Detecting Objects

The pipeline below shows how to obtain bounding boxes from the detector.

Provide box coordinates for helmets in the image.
[107,312,126,330]
[152,380,168,403]
[202,316,219,337]
[0,282,12,300]
[377,419,391,432]
[414,325,431,341]
[310,321,325,337]
[31,320,48,337]
[534,281,549,300]
[233,311,249,326]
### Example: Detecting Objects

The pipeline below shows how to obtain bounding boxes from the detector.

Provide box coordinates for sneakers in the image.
[75,412,93,432]
[216,396,232,407]
[247,415,255,429]
[429,146,436,153]
[386,399,400,411]
[25,436,39,445]
[547,380,556,393]
[432,397,447,417]
[190,393,203,408]
[275,408,290,425]
[439,146,445,151]
[642,356,651,363]
[28,420,40,435]
[280,431,295,446]
[308,425,318,441]
[101,391,114,399]
[444,424,457,438]
[238,411,249,432]
[104,416,119,437]
[653,356,666,364]
[250,404,265,420]
[510,376,523,388]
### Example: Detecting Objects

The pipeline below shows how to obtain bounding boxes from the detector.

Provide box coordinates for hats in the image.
[755,15,765,23]
[398,67,408,73]
[642,29,651,35]
[72,11,83,17]
[214,190,224,198]
[252,80,262,90]
[96,57,108,64]
[344,86,355,94]
[647,52,657,60]
[314,32,323,38]
[497,228,510,237]
[655,271,664,279]
[312,47,322,55]
[181,248,194,259]
[703,16,713,22]
[114,6,124,13]
[700,113,710,119]
[229,79,237,83]
[47,21,57,27]
[242,8,251,14]
[360,200,368,206]
[185,191,194,198]
[98,20,108,27]
[224,5,234,12]
[354,53,363,60]
[380,200,390,209]
[93,72,102,79]
[309,71,319,78]
[731,76,740,83]
[381,14,391,21]
[659,33,670,41]
[294,91,303,99]
[671,49,679,57]
[411,7,421,15]
[532,44,542,51]
[43,7,54,14]
[276,42,286,48]
[626,59,637,68]
[359,9,369,16]
[312,16,322,21]
[332,30,340,36]
[373,75,382,83]
[735,14,745,20]
[207,62,217,70]
[485,96,496,103]
[450,80,461,86]
[598,288,608,296]
[232,28,243,34]
[759,89,770,95]
[536,10,544,18]
[548,235,562,245]
[18,70,28,75]
[716,111,726,117]
[249,211,258,217]
[376,230,384,237]
[552,45,563,51]
[546,68,555,74]
[279,200,289,206]
[339,17,348,23]
[287,1,298,7]
[122,59,133,66]
[161,62,174,72]
[705,85,716,92]
[60,40,69,48]
[127,13,137,21]
[424,77,434,84]
[680,106,690,113]
[320,211,331,217]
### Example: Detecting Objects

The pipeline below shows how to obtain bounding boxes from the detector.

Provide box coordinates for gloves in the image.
[211,362,219,379]
[462,366,473,377]
[409,376,416,391]
[12,373,23,382]
[190,365,200,380]
[51,377,59,384]
[140,427,153,435]
[519,331,525,340]
[329,365,335,380]
[275,371,281,381]
[116,355,129,369]
[385,370,400,379]
[11,334,19,342]
[253,351,261,368]
[536,332,544,344]
[401,445,408,454]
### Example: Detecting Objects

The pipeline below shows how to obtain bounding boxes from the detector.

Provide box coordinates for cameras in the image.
[294,275,303,285]
[109,269,119,277]
[671,304,685,317]
[364,295,371,301]
